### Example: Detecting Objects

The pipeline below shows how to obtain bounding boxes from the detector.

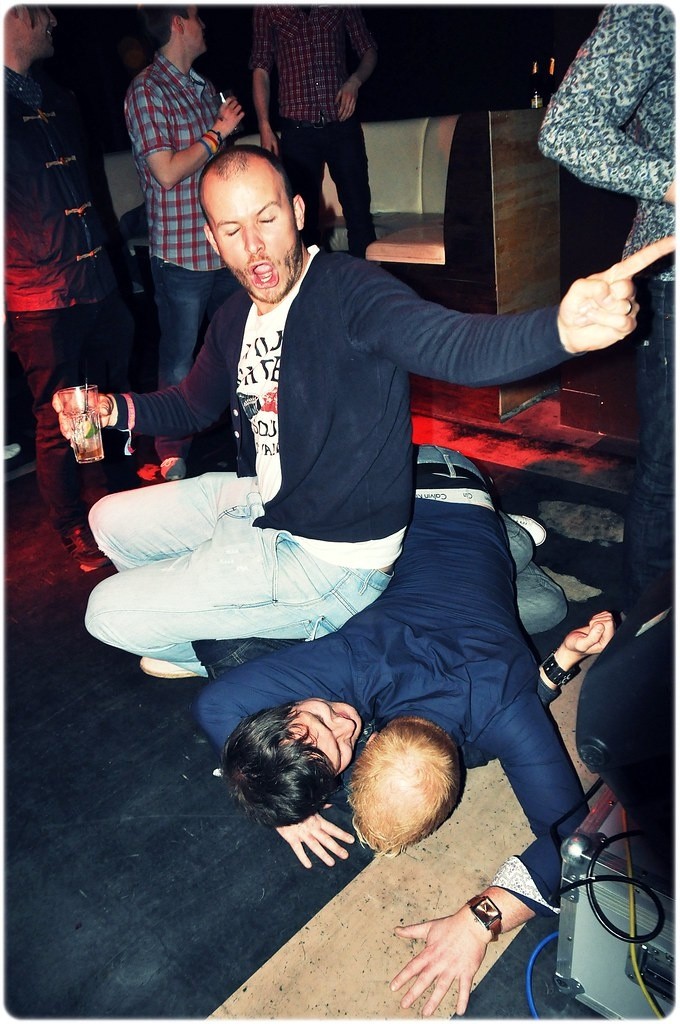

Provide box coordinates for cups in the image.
[212,89,245,135]
[57,384,105,463]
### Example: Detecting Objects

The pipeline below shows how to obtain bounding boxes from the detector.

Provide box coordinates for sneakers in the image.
[59,521,110,567]
[159,456,187,481]
[140,655,209,679]
[505,512,547,547]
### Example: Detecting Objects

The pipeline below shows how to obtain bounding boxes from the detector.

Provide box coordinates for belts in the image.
[292,119,325,129]
[415,462,487,492]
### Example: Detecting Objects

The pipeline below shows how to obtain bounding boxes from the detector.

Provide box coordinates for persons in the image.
[537,5,674,595]
[249,5,378,259]
[52,145,674,679]
[123,5,244,481]
[197,443,615,1018]
[6,6,132,568]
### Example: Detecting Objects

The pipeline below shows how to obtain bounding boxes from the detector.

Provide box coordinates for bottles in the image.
[531,88,543,109]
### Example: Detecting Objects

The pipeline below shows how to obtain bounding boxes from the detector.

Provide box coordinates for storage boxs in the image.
[554,787,675,1020]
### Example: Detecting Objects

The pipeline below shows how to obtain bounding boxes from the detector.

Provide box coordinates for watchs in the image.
[466,895,503,942]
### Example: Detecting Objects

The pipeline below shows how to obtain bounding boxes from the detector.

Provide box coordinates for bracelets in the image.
[118,393,136,456]
[196,129,223,162]
[542,650,575,686]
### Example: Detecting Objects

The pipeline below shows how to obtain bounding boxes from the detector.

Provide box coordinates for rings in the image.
[624,298,634,317]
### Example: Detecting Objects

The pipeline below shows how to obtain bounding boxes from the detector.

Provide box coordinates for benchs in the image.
[107,104,561,421]
